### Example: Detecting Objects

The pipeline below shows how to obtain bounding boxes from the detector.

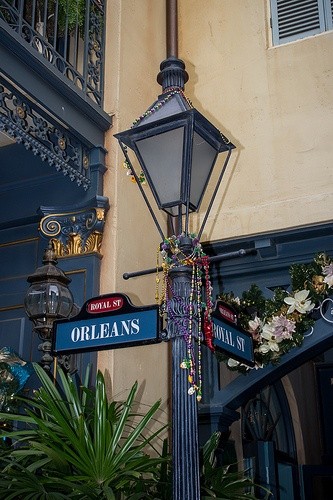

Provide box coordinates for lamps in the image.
[24,240,78,375]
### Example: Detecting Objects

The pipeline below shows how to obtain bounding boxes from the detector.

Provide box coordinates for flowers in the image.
[214,251,333,373]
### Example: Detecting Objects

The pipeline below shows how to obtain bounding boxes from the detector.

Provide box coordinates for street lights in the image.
[113,56,246,500]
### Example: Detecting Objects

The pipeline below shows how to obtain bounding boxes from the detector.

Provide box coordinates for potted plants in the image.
[34,0,87,54]
